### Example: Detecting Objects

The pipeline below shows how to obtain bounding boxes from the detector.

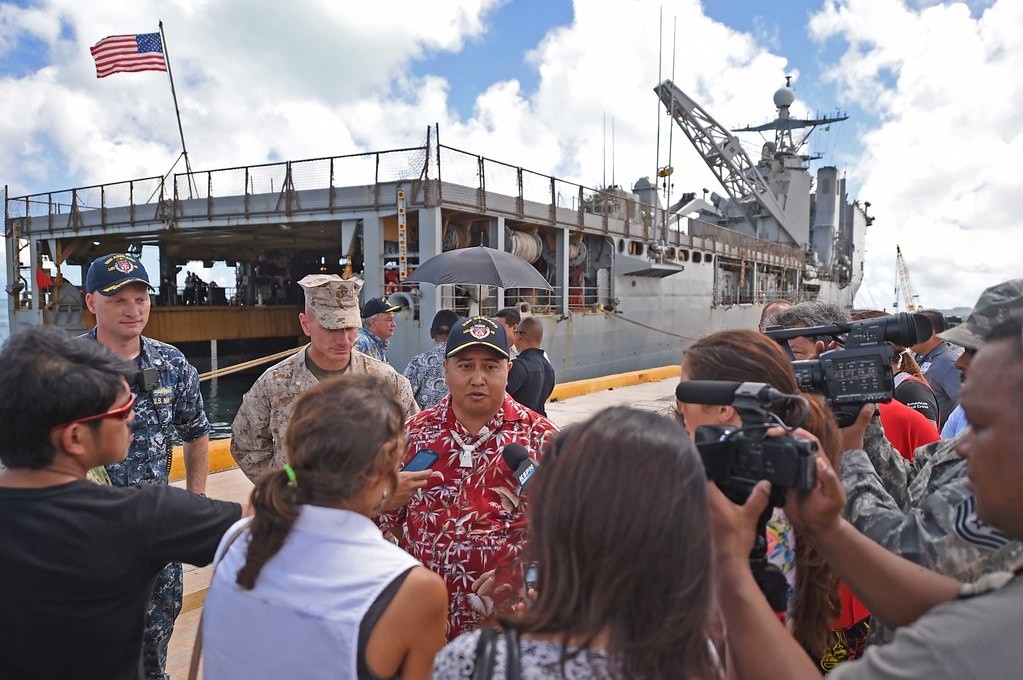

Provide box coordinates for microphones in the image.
[502,443,536,487]
[676,379,796,410]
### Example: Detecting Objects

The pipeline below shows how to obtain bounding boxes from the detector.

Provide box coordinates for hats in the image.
[935,277,1023,349]
[432,309,460,335]
[363,297,402,318]
[86,253,156,296]
[447,315,509,358]
[296,274,366,329]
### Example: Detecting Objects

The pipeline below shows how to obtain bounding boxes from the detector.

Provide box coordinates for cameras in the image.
[692,424,818,490]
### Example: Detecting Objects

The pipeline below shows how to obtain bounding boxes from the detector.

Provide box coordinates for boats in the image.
[0,5,876,401]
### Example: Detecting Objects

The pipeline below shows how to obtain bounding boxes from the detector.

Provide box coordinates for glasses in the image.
[514,331,527,335]
[52,393,136,432]
[964,347,977,356]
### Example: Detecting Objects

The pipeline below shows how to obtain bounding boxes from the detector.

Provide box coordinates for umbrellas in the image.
[400,244,554,316]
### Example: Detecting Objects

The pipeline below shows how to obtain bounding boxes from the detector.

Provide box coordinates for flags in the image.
[89,32,168,78]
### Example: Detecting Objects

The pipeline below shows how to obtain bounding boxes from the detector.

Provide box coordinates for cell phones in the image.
[400,448,439,471]
[521,561,539,599]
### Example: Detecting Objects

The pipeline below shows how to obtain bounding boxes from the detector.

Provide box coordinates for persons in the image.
[73,254,212,680]
[230,273,422,488]
[372,317,562,648]
[653,277,1023,680]
[352,297,556,419]
[432,405,732,680]
[160,271,216,305]
[0,324,433,680]
[386,263,414,295]
[203,373,447,680]
